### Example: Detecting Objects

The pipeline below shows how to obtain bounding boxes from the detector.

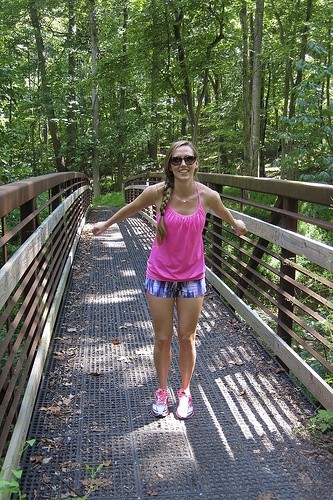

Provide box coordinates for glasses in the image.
[169,155,198,166]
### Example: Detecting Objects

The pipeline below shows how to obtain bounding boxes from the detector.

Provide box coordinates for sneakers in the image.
[151,386,170,417]
[176,388,194,419]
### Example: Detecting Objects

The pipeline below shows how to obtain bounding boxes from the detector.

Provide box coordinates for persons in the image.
[92,139,248,420]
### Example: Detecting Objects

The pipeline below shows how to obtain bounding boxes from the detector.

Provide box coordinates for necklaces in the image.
[182,198,188,204]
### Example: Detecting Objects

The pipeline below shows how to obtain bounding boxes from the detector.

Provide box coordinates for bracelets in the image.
[233,223,240,230]
